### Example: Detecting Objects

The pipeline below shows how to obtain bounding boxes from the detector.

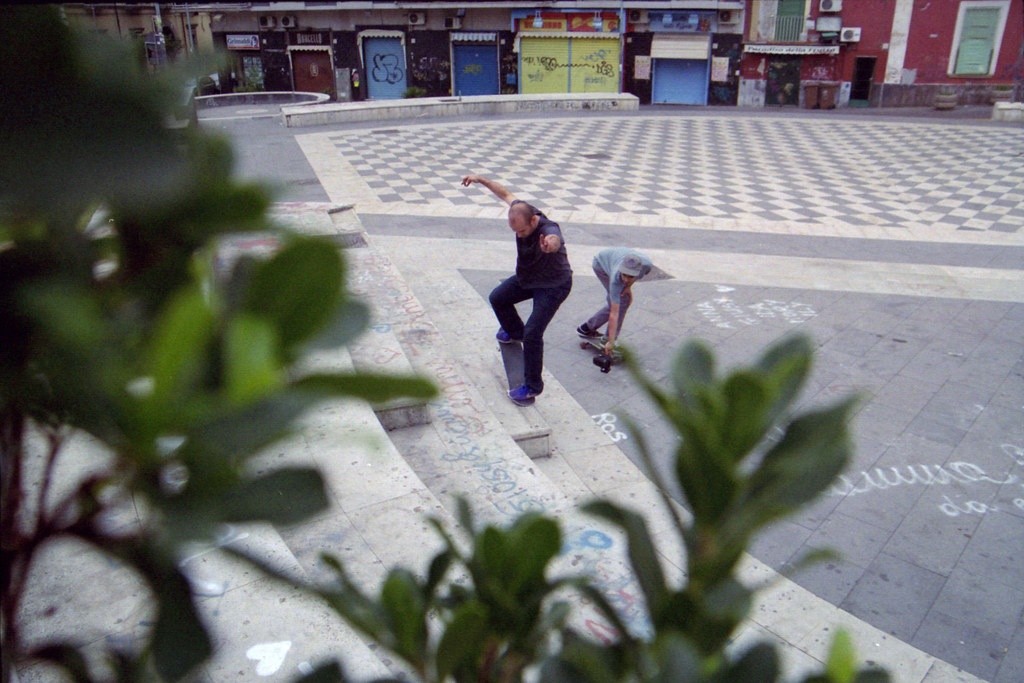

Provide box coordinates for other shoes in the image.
[600,337,623,353]
[576,325,604,338]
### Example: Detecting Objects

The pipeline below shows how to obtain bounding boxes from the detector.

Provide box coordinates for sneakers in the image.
[496,331,524,343]
[509,384,542,401]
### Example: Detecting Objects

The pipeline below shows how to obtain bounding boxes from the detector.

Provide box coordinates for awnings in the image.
[650,35,710,59]
[354,25,406,45]
[286,45,333,70]
[744,41,839,54]
[451,33,496,42]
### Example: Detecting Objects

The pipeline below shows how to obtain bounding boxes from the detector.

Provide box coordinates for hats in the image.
[621,254,641,276]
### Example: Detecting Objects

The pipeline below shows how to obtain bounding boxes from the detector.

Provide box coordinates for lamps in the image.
[591,11,604,29]
[532,10,545,28]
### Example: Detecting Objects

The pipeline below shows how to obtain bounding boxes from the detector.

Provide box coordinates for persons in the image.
[461,176,573,401]
[577,247,651,356]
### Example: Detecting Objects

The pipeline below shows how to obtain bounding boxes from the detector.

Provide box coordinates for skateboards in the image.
[577,332,625,360]
[497,327,536,406]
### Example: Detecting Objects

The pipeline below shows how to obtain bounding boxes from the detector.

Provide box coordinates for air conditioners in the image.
[280,16,297,29]
[443,17,462,30]
[718,9,741,25]
[818,0,843,12]
[408,12,426,26]
[259,15,276,28]
[628,10,650,24]
[839,26,862,43]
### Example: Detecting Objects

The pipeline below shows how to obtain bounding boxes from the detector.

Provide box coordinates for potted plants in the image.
[989,82,1015,104]
[931,87,959,110]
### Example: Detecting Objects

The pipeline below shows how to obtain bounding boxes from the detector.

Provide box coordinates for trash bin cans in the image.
[803,80,839,110]
